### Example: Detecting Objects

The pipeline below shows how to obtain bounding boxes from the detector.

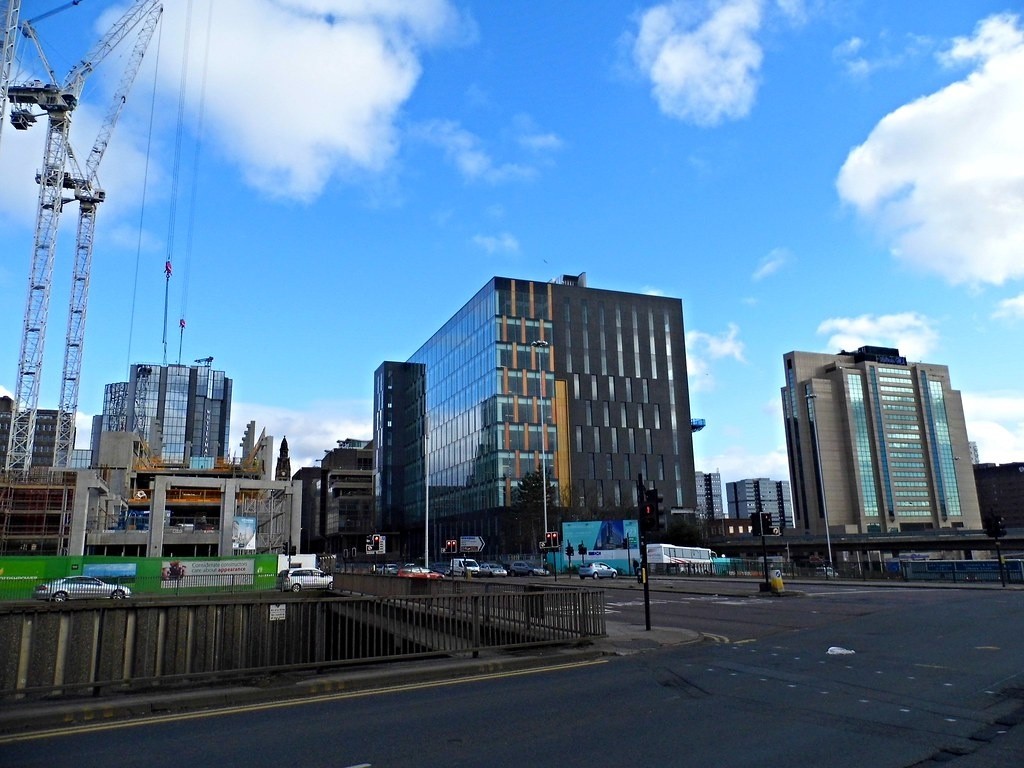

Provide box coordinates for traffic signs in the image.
[460,536,486,553]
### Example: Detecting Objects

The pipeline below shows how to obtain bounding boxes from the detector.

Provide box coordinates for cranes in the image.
[0,1,268,476]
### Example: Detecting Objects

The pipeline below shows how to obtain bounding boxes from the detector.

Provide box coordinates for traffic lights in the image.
[622,538,627,549]
[545,531,559,548]
[641,488,665,531]
[982,515,1007,537]
[283,542,296,556]
[565,547,574,556]
[750,512,772,536]
[372,534,380,550]
[446,540,457,553]
[578,544,587,555]
[342,547,357,560]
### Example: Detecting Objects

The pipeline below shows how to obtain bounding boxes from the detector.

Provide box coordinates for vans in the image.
[450,558,480,577]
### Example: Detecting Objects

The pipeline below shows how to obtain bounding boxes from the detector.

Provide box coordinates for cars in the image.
[32,576,132,603]
[480,563,507,577]
[509,561,551,576]
[816,567,839,578]
[374,563,450,580]
[579,562,618,579]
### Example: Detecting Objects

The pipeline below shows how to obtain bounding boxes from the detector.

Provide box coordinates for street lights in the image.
[805,394,832,567]
[301,528,310,553]
[531,340,548,548]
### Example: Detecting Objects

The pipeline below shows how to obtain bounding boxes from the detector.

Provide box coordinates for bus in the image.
[646,543,717,575]
